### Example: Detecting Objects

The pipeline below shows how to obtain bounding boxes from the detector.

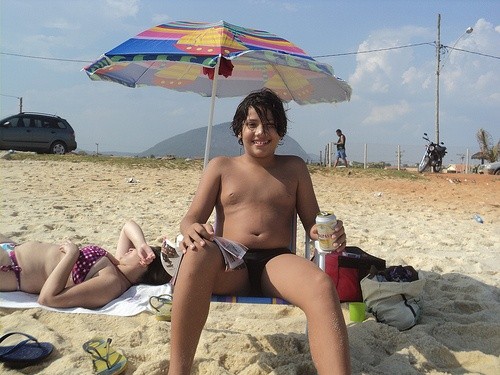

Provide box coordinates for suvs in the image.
[0,112,77,155]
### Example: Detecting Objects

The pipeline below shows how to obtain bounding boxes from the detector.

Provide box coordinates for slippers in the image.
[149,293,173,321]
[81,337,128,375]
[0,332,55,364]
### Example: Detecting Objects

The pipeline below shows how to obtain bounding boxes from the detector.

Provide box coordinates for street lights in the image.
[436,27,473,144]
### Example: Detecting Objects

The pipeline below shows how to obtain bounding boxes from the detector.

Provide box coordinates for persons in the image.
[167,87,351,375]
[0,220,181,311]
[332,129,348,168]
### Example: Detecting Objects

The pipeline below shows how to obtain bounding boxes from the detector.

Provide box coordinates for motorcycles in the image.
[418,132,447,173]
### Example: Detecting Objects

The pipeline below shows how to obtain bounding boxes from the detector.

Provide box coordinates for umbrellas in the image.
[84,19,352,167]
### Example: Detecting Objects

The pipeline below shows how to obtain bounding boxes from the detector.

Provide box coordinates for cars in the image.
[477,156,500,175]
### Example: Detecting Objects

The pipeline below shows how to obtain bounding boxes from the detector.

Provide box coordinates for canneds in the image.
[316,211,340,252]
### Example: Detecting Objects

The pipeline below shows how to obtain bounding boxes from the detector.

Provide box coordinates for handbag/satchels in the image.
[325,245,387,303]
[359,265,424,331]
